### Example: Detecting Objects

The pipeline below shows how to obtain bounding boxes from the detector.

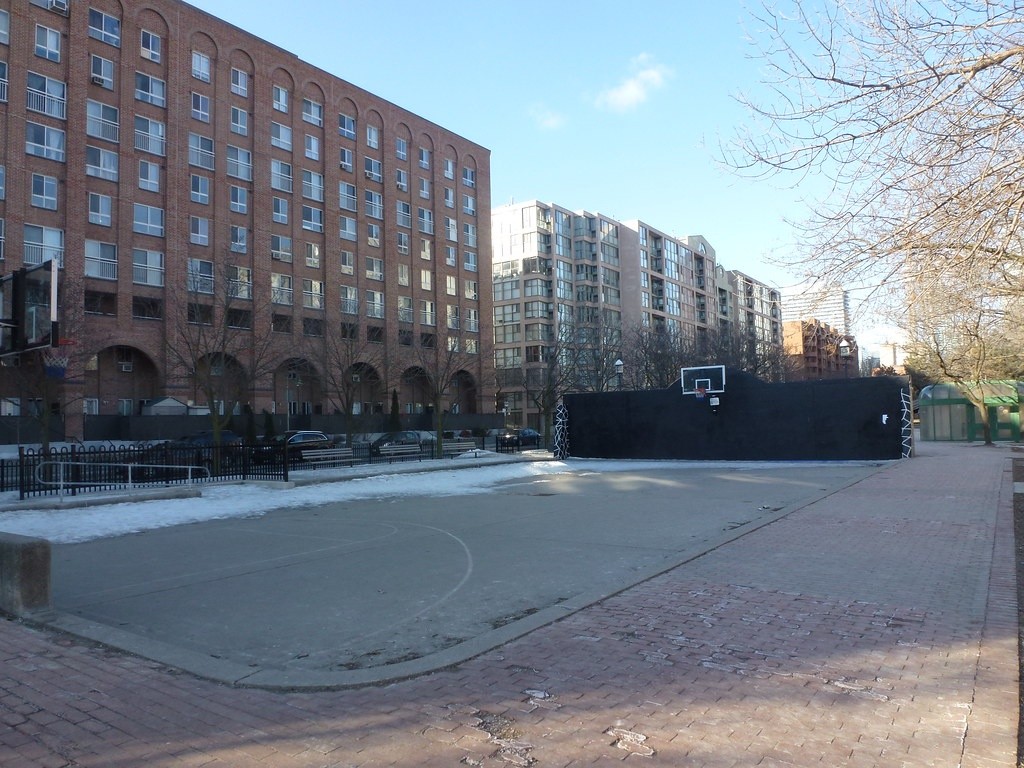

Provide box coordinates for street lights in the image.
[287,371,305,429]
[504,400,508,429]
[615,359,624,390]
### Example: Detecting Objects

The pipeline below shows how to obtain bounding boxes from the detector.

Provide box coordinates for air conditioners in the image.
[340,163,346,169]
[365,172,373,178]
[50,0,66,12]
[272,252,282,259]
[118,365,133,372]
[92,76,104,84]
[398,183,403,190]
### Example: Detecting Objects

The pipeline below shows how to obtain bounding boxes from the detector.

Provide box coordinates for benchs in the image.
[379,444,425,464]
[301,448,362,470]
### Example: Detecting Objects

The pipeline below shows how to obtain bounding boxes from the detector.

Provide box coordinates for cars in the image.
[117,442,213,481]
[180,430,242,466]
[501,429,541,446]
[370,430,437,456]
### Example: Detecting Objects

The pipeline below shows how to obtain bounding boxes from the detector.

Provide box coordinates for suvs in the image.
[250,430,336,467]
